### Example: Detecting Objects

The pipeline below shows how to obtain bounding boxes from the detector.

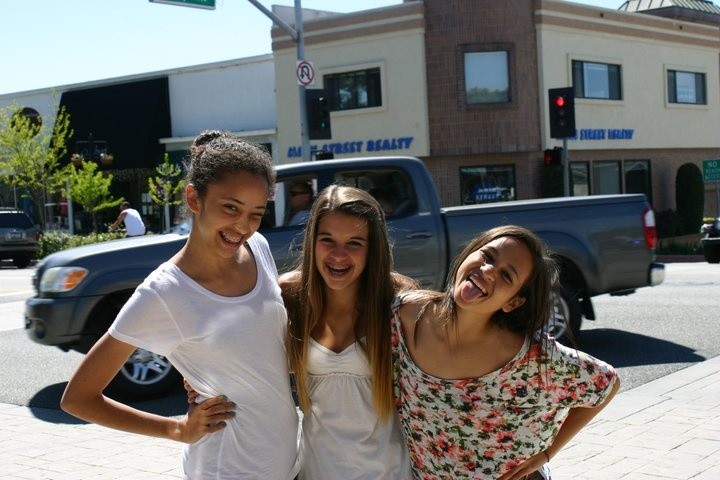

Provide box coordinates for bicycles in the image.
[110,224,127,238]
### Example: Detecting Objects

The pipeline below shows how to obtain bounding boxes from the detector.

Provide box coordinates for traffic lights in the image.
[548,87,577,138]
[544,148,560,167]
[305,88,331,140]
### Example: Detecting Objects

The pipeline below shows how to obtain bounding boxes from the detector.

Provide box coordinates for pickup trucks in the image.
[27,156,667,400]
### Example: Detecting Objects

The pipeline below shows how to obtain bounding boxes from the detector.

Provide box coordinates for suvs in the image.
[1,208,40,268]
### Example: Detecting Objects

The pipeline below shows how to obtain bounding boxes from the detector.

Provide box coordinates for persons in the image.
[59,134,299,480]
[284,181,315,227]
[387,224,621,480]
[183,184,419,480]
[110,201,146,237]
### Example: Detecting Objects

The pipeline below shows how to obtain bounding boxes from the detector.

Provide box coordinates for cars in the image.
[701,218,720,263]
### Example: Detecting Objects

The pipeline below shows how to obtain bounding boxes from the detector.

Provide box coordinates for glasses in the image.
[287,190,308,196]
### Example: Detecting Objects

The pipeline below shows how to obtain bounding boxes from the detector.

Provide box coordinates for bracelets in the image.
[544,450,550,464]
